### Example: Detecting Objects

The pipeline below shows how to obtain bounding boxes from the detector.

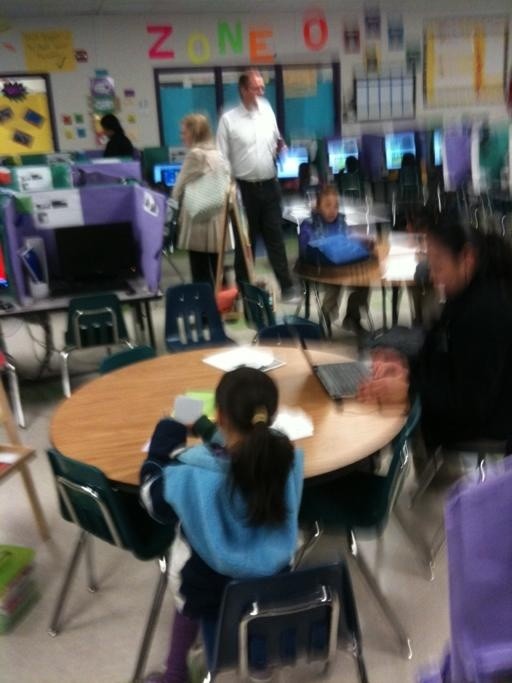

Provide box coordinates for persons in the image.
[168,109,236,294]
[333,154,376,199]
[388,151,423,231]
[215,66,308,302]
[291,161,320,191]
[136,364,305,682]
[295,185,372,339]
[99,112,135,157]
[350,217,510,459]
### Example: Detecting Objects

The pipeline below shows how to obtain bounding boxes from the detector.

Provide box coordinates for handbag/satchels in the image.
[183,170,226,220]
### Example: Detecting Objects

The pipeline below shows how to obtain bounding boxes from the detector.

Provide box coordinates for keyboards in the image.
[48,279,135,296]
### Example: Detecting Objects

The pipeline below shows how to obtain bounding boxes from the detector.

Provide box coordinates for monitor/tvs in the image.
[152,162,182,187]
[275,145,309,182]
[323,137,360,177]
[430,127,443,168]
[54,220,139,280]
[385,130,417,171]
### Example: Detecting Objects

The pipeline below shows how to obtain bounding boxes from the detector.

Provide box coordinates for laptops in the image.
[282,315,372,404]
